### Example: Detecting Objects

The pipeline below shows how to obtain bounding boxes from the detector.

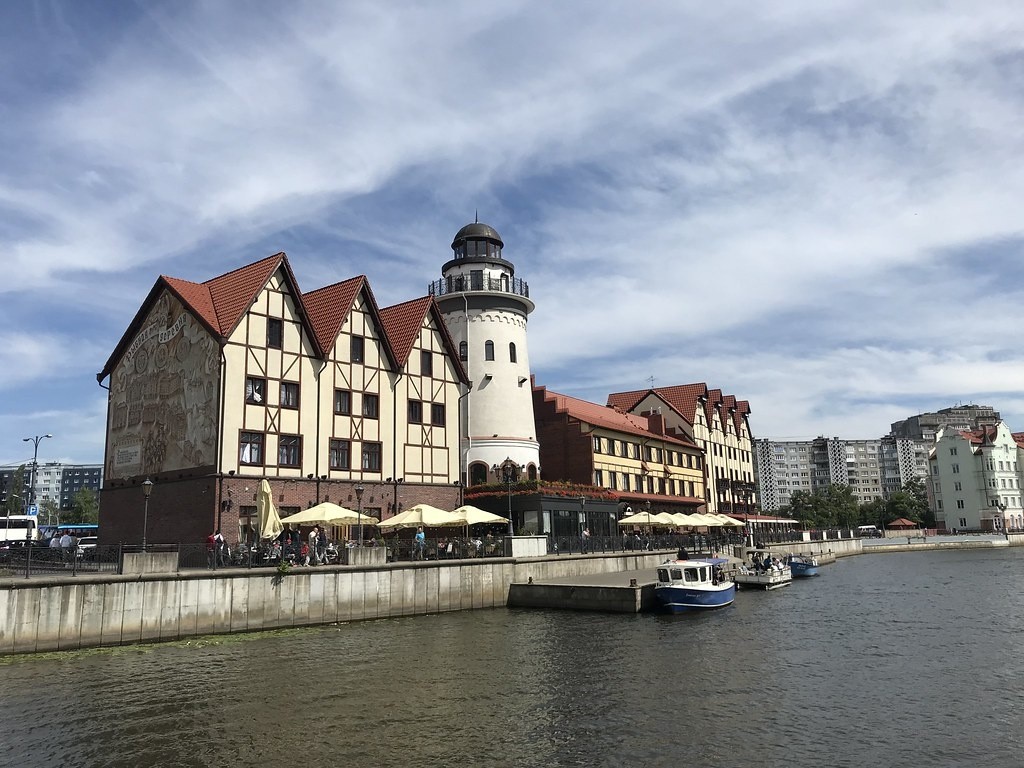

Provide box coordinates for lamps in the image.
[229,470,235,476]
[519,377,527,382]
[387,478,391,482]
[398,478,403,482]
[486,373,492,379]
[308,474,313,479]
[454,481,458,486]
[322,475,328,480]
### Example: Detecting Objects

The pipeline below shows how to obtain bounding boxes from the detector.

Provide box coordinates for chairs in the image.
[444,543,455,560]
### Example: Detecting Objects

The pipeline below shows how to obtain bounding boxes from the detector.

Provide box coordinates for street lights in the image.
[355,484,364,547]
[23,434,52,515]
[579,494,586,554]
[646,500,653,551]
[141,476,154,553]
[494,457,522,536]
[753,506,759,543]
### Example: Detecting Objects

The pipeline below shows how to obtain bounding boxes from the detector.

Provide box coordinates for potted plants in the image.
[482,540,491,553]
[494,543,504,556]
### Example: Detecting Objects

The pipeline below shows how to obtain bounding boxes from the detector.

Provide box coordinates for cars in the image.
[857,525,883,538]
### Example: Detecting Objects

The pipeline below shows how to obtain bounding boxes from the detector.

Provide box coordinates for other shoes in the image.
[317,562,324,565]
[304,563,310,566]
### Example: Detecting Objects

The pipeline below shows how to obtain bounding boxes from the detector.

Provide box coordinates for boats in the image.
[735,549,792,583]
[654,557,735,614]
[780,555,818,576]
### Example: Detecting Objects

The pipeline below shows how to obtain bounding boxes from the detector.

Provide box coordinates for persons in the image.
[665,526,670,535]
[582,527,590,554]
[370,538,379,547]
[278,523,301,543]
[769,528,795,541]
[677,546,690,560]
[733,564,736,569]
[949,526,957,536]
[414,527,425,556]
[206,530,225,569]
[304,528,329,566]
[718,571,725,582]
[742,528,749,547]
[753,553,784,570]
[635,535,640,540]
[348,540,357,548]
[49,531,78,560]
[646,533,649,551]
[756,541,764,561]
[925,526,928,537]
[671,529,674,533]
[742,562,747,571]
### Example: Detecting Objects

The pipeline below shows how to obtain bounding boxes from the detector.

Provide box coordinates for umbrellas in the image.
[281,502,379,539]
[376,504,509,537]
[257,479,284,541]
[617,511,746,536]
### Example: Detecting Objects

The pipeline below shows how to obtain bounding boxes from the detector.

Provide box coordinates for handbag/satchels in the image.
[216,535,221,543]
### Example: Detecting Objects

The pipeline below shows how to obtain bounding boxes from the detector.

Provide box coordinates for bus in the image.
[0,515,98,561]
